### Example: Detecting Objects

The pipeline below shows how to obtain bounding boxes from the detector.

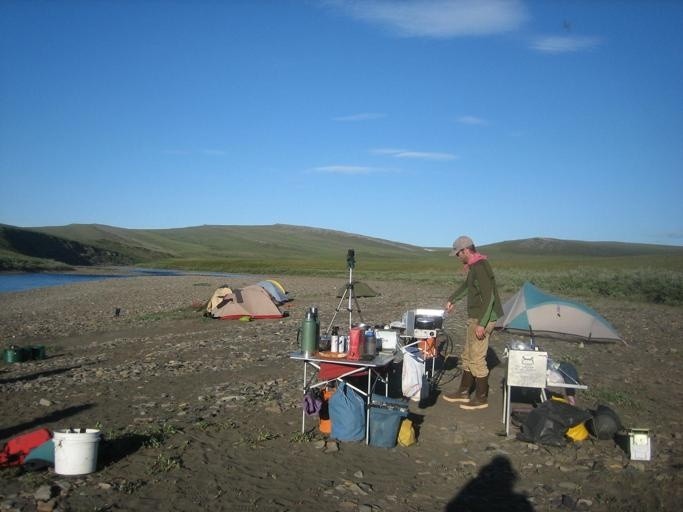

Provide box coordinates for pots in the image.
[416,317,435,325]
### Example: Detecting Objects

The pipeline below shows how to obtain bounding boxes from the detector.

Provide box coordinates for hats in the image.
[447,236,472,257]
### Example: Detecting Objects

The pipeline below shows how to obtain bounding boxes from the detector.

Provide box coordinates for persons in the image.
[441,235,504,410]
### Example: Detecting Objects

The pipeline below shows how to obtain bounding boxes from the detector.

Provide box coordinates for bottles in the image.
[350,327,377,360]
[297,308,320,357]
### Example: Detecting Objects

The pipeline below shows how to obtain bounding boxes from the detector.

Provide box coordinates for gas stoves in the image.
[413,325,441,339]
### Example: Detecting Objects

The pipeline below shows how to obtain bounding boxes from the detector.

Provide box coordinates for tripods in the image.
[324,284,364,334]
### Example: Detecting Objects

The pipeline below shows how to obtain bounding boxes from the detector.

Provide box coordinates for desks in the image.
[290,348,395,446]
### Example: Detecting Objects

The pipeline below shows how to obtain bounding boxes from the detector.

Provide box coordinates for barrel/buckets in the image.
[53,429,101,476]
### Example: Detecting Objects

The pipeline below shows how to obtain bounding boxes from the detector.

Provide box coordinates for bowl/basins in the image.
[318,336,331,352]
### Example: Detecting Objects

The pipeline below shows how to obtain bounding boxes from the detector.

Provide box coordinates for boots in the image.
[442,370,474,403]
[458,376,488,409]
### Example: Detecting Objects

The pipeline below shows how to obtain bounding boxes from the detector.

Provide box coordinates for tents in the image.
[336,281,377,298]
[494,278,628,345]
[256,278,289,304]
[206,284,283,320]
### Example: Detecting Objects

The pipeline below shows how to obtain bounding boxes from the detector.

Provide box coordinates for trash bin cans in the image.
[369,393,409,448]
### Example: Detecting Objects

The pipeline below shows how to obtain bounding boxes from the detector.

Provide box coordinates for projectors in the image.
[414,329,437,338]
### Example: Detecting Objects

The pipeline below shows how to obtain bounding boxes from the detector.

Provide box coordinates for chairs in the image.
[396,335,436,379]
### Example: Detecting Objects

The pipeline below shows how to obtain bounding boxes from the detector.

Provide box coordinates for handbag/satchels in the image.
[584,399,622,441]
[515,399,593,450]
